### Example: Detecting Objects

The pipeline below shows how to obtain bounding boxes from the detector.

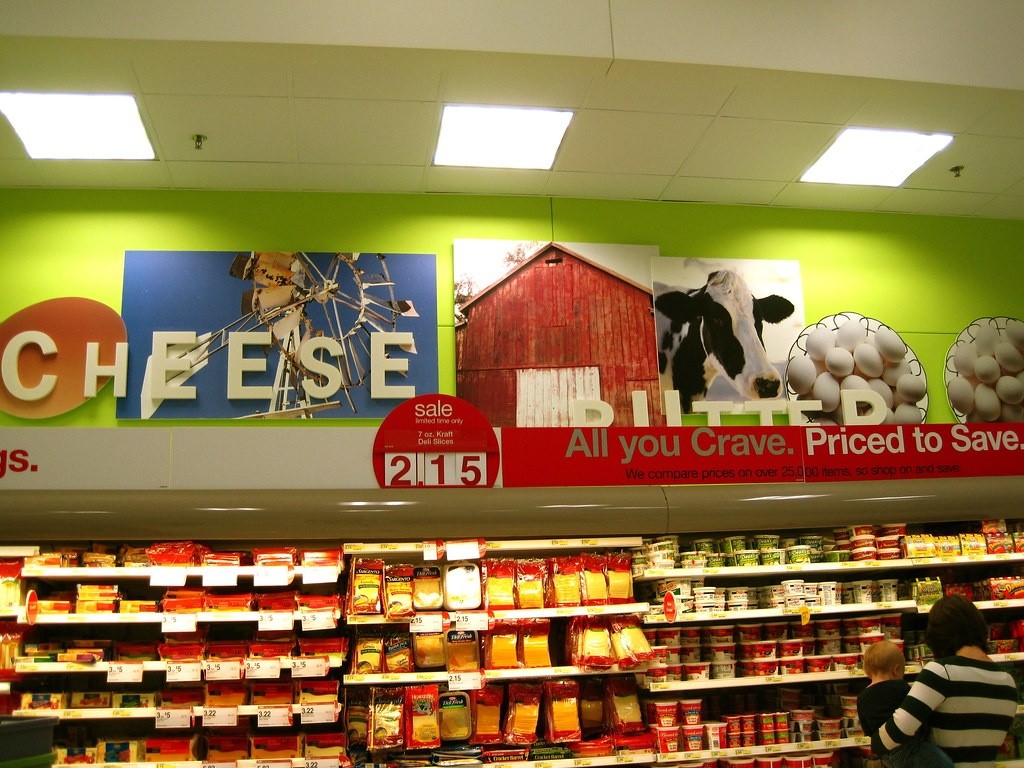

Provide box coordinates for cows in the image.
[652,270,795,415]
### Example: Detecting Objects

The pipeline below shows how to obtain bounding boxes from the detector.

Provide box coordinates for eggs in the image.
[948,322,1024,423]
[787,319,927,426]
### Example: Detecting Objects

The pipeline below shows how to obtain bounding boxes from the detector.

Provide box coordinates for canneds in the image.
[633,523,905,690]
[640,697,727,754]
[719,682,862,748]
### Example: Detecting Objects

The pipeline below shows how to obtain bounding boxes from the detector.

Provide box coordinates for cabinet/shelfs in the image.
[0,545,42,717]
[11,557,347,768]
[632,551,1024,768]
[342,535,658,768]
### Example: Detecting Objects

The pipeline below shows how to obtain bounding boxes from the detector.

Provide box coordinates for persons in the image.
[869,594,1018,762]
[857,642,953,768]
